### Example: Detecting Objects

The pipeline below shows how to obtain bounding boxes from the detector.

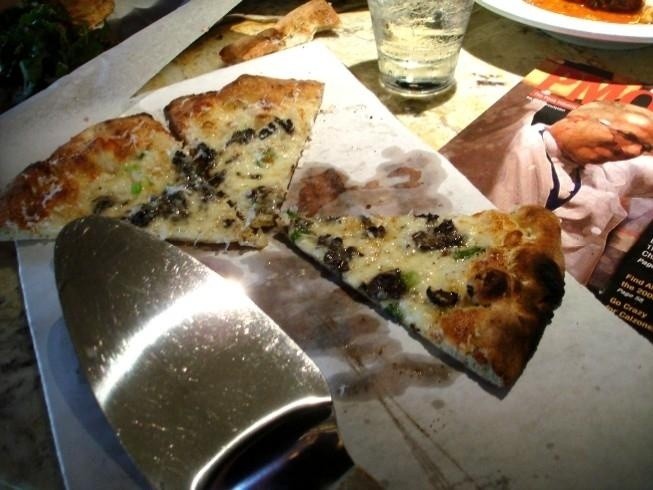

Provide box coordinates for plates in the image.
[476,0,653,51]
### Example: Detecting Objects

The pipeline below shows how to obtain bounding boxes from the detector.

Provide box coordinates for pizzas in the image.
[0,111,267,250]
[166,75,323,230]
[219,1,340,64]
[272,205,565,394]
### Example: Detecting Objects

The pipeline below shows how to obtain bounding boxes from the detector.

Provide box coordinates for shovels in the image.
[53,215,384,490]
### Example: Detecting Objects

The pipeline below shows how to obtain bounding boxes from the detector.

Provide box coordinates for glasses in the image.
[599,118,653,156]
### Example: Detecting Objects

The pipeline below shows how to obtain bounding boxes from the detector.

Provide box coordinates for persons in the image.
[439,79,652,281]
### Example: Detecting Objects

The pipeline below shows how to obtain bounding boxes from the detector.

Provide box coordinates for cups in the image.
[366,0,475,100]
